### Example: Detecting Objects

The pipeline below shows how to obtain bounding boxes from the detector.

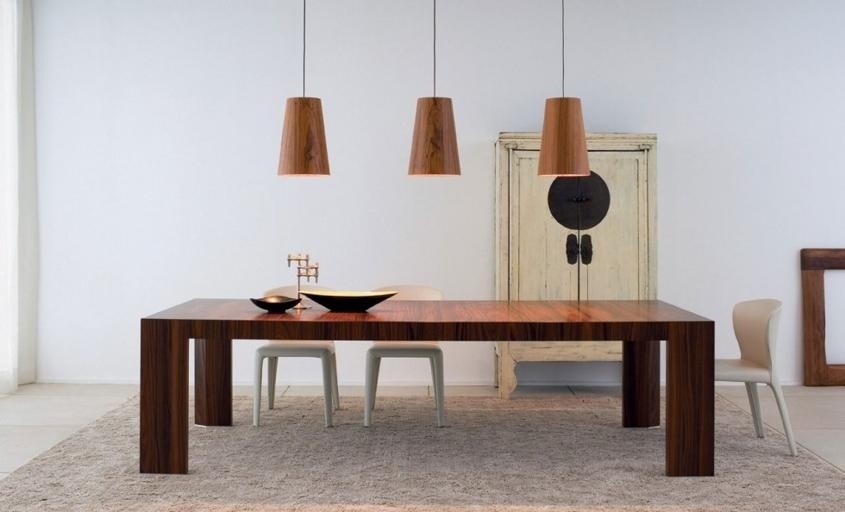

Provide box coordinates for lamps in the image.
[278,0,331,178]
[536,0,591,176]
[407,1,462,179]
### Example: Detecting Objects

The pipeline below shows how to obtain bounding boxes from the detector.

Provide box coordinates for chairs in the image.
[362,287,448,428]
[252,286,342,429]
[714,298,799,458]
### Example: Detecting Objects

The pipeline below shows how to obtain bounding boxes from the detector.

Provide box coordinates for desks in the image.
[138,298,716,476]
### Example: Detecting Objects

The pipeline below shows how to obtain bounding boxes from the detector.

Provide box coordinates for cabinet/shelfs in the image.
[494,130,660,399]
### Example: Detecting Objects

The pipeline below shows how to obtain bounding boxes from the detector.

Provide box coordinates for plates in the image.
[248,295,304,313]
[297,289,401,313]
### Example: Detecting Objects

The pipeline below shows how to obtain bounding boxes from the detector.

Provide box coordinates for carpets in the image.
[0,388,844,512]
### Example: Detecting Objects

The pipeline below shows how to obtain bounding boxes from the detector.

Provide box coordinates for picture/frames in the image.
[802,247,845,387]
